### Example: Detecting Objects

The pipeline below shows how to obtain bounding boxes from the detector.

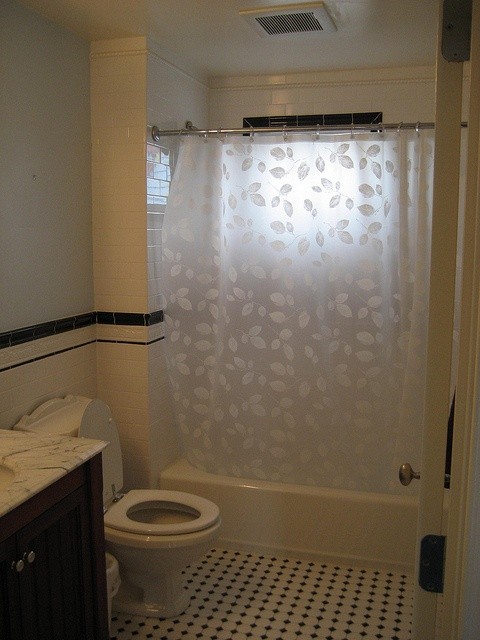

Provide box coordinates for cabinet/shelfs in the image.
[0,450,108,640]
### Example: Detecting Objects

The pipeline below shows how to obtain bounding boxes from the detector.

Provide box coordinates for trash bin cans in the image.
[105,553,121,634]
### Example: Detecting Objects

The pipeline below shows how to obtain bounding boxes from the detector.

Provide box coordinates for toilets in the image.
[12,394,219,618]
